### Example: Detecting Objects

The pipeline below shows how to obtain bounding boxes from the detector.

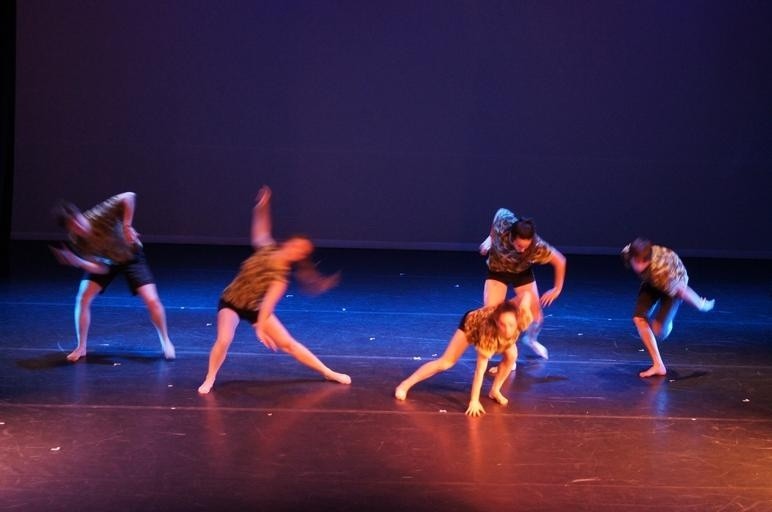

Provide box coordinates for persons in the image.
[621,236,720,378]
[44,190,180,363]
[477,204,567,374]
[394,289,533,419]
[196,185,352,397]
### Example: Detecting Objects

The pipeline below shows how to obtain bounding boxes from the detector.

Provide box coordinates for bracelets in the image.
[121,223,134,230]
